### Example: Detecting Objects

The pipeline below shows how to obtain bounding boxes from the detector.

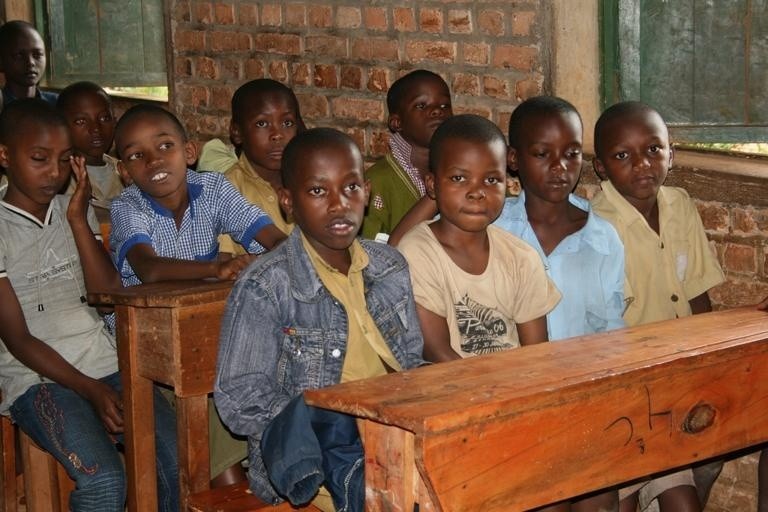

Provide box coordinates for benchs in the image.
[186,477,325,510]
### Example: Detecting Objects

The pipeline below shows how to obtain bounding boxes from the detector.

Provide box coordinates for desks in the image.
[301,305,766,512]
[87,273,239,511]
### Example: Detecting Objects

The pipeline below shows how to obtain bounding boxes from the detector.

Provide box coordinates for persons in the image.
[360,70,451,245]
[217,78,305,260]
[57,81,127,213]
[435,94,651,511]
[213,125,433,512]
[588,101,726,511]
[2,97,178,511]
[396,114,570,512]
[1,20,59,123]
[104,103,288,488]
[196,138,242,175]
